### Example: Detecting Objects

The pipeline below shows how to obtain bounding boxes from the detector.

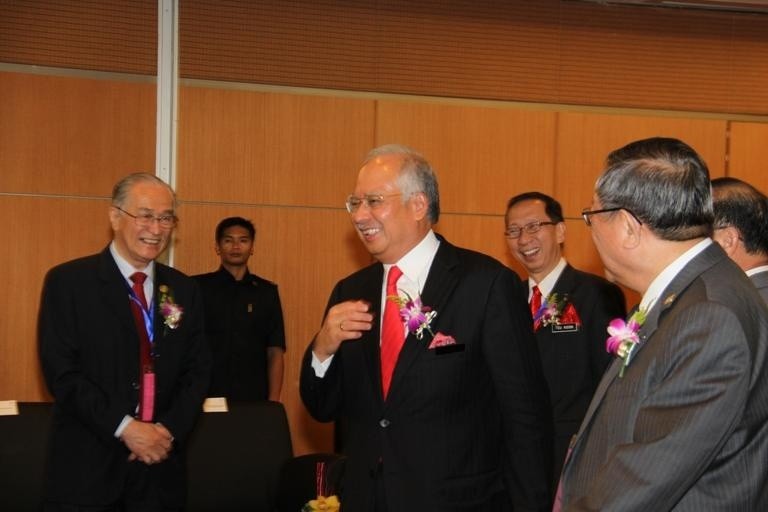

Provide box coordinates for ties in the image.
[129,272,158,423]
[380,266,407,401]
[529,286,542,333]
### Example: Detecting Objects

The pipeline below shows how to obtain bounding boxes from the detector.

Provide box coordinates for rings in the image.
[340,321,346,331]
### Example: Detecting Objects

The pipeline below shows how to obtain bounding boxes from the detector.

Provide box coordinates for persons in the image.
[706,175,768,307]
[297,144,555,511]
[502,191,628,478]
[35,173,218,511]
[550,135,768,512]
[188,216,285,404]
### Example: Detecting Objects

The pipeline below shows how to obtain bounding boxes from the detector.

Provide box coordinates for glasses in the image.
[118,207,178,229]
[503,220,555,238]
[581,207,619,227]
[345,193,402,212]
